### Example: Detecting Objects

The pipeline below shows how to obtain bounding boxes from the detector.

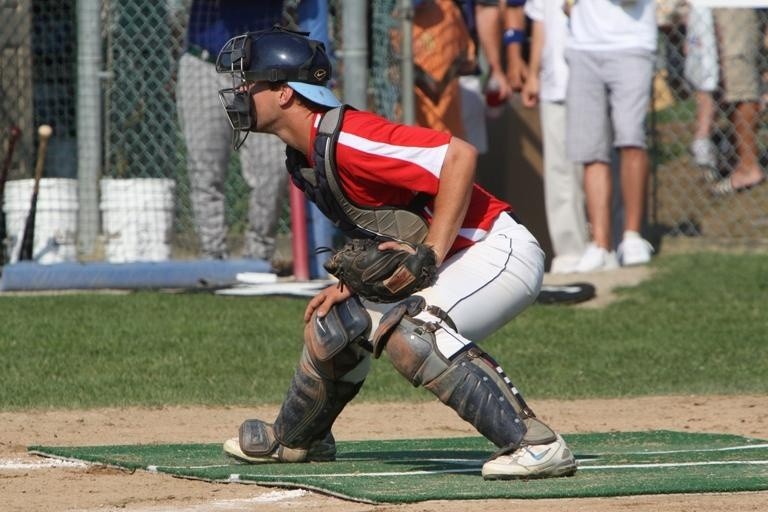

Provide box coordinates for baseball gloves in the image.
[322,237,438,303]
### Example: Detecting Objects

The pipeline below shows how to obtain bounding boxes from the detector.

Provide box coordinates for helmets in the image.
[216,28,344,152]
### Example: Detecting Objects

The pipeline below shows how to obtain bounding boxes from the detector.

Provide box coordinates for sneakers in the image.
[573,243,620,273]
[479,429,578,481]
[618,230,654,266]
[692,139,720,168]
[222,432,339,465]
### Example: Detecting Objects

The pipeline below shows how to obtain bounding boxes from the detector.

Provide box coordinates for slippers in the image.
[708,176,765,197]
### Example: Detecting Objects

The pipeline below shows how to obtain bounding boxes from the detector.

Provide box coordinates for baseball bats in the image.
[0,125,52,262]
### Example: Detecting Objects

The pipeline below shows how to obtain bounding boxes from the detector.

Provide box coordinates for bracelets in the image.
[503,30,523,47]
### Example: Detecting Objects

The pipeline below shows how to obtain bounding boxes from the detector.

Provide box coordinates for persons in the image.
[215,29,577,481]
[524,0,587,274]
[175,4,294,264]
[562,0,660,272]
[657,0,768,193]
[392,0,511,139]
[504,0,530,90]
